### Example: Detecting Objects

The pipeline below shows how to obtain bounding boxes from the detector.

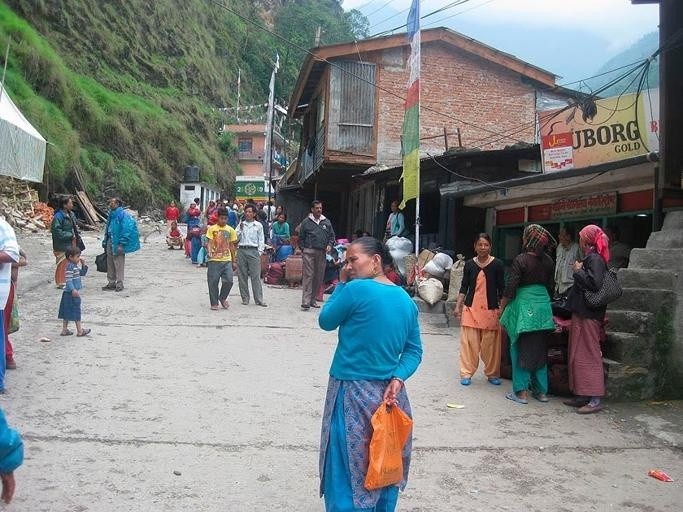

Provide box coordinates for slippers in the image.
[77,328,90,336]
[507,391,528,403]
[61,330,72,337]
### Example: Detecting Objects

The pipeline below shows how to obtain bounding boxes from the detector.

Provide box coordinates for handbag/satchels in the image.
[75,237,85,251]
[584,268,622,307]
[96,252,107,273]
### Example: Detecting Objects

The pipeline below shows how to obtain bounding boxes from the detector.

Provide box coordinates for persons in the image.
[0,406,24,504]
[317,233,424,512]
[0,216,21,396]
[381,200,406,248]
[497,222,557,404]
[102,197,127,292]
[164,195,371,311]
[560,224,611,414]
[553,229,579,298]
[453,231,504,386]
[55,247,91,337]
[50,196,81,287]
[4,243,28,370]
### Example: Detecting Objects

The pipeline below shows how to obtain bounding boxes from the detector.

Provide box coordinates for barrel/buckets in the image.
[278,244,293,261]
[285,254,302,283]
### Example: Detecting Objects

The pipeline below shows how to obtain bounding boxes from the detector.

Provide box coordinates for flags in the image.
[399,0,420,202]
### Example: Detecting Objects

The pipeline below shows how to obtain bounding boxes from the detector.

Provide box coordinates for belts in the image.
[240,246,255,248]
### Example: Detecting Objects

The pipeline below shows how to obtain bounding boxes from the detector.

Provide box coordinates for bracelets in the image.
[392,377,404,387]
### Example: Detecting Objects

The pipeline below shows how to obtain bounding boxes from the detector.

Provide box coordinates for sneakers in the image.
[212,304,219,311]
[577,403,603,414]
[489,376,502,386]
[563,398,588,408]
[220,296,229,310]
[302,304,311,309]
[101,285,116,291]
[311,302,320,308]
[461,378,471,385]
